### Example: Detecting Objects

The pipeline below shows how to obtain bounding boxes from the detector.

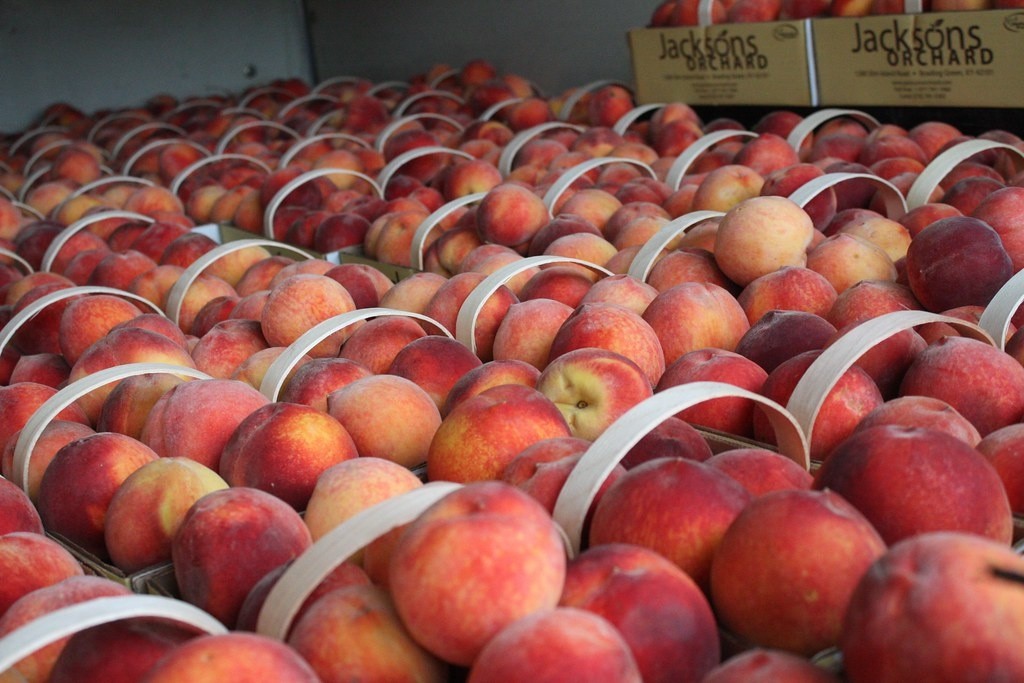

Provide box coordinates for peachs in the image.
[1,61,1024,683]
